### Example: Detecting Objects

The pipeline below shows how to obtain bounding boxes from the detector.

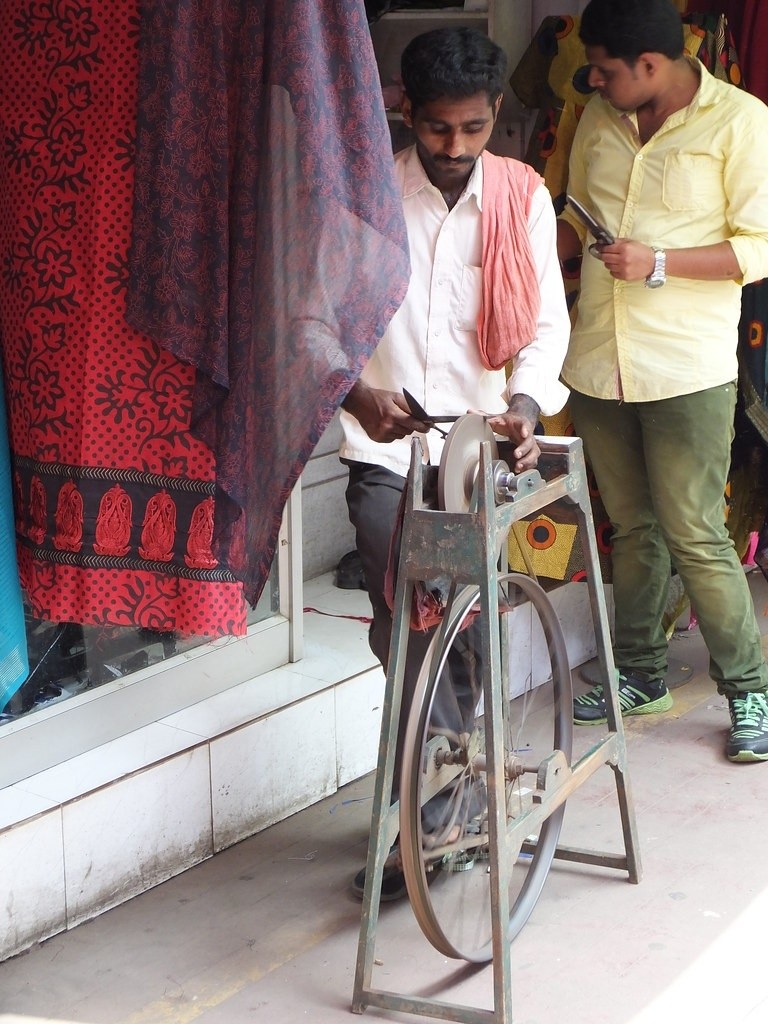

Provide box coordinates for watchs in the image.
[646,248,667,289]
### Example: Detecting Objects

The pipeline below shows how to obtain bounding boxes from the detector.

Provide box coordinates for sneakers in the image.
[727,690,768,762]
[573,671,674,725]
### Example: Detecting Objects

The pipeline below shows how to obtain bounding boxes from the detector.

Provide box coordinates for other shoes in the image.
[351,844,428,901]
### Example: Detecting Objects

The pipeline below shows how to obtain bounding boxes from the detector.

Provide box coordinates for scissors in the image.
[565,193,611,261]
[399,387,498,441]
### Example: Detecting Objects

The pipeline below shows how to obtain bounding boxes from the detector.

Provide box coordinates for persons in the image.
[286,26,571,903]
[558,0,768,762]
